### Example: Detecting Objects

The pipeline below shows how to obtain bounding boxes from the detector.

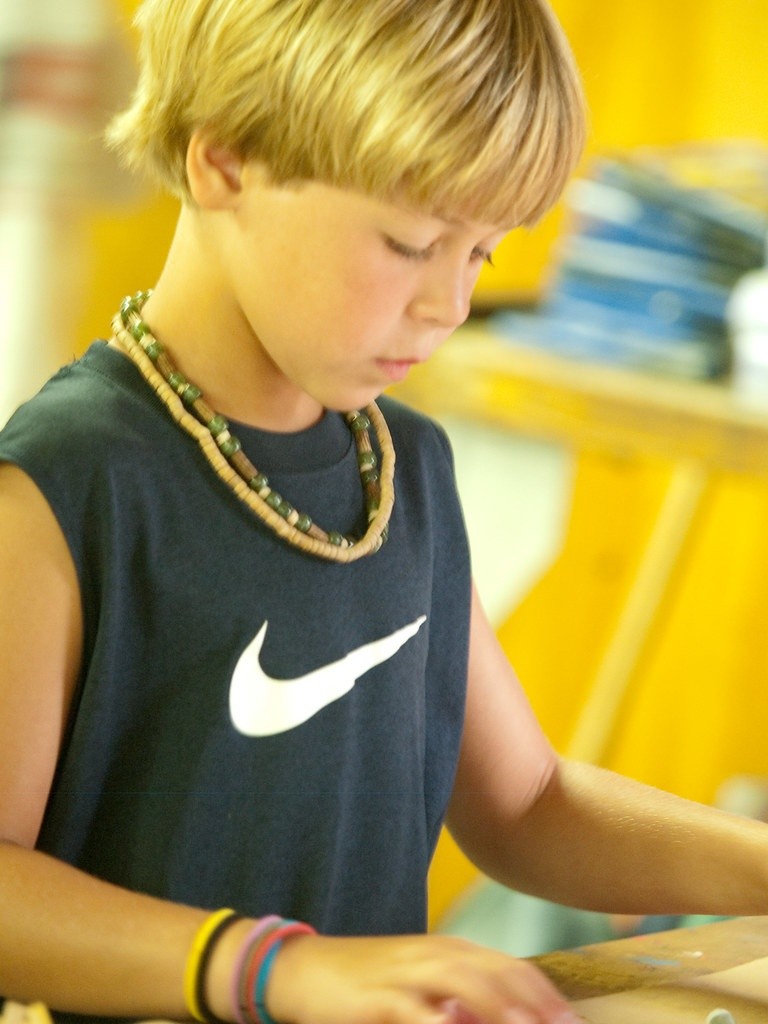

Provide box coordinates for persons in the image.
[3,2,768,1023]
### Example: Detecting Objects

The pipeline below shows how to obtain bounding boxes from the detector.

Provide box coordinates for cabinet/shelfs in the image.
[403,331,767,938]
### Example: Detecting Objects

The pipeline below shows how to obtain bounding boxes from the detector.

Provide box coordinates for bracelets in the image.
[230,914,317,1023]
[185,907,241,1023]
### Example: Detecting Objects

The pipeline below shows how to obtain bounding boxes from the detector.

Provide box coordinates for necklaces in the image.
[111,289,398,562]
